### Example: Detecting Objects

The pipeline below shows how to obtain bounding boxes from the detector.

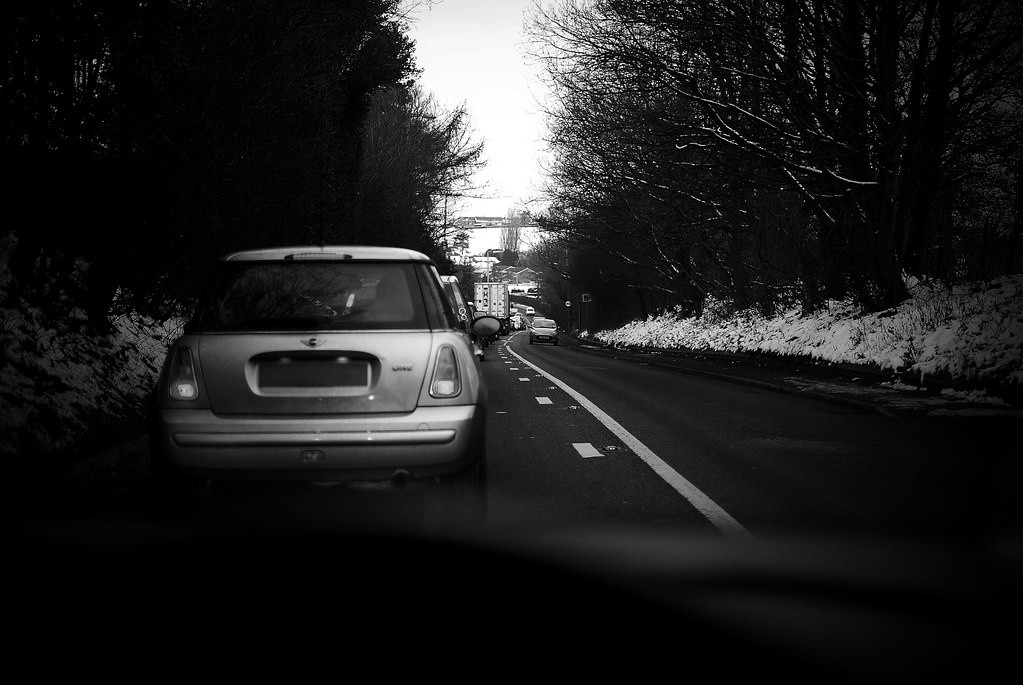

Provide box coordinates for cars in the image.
[528,318,561,346]
[153,245,503,482]
[438,273,536,334]
